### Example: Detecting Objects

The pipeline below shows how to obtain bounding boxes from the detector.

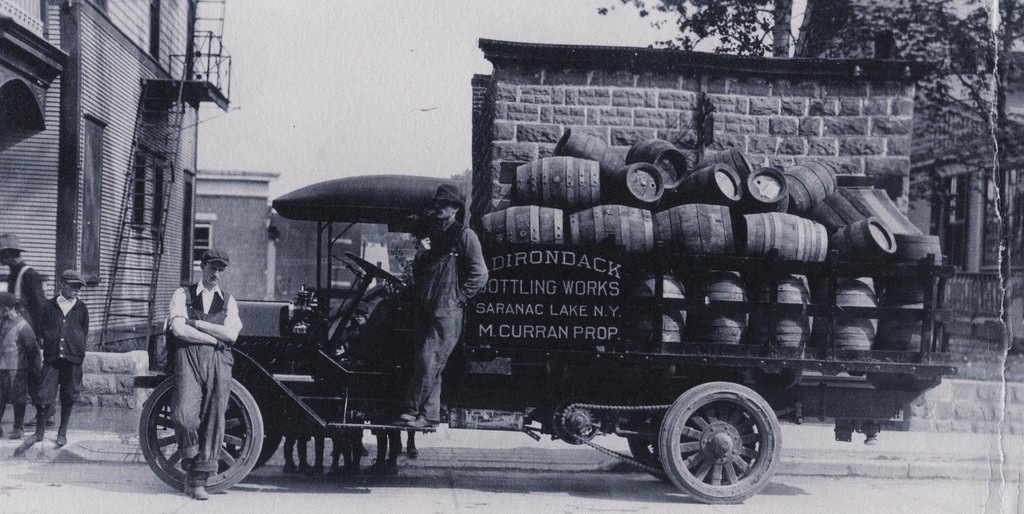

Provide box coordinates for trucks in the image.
[130,169,960,508]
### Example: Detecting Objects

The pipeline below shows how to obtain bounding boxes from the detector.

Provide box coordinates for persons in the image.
[390,183,489,429]
[0,232,55,425]
[279,290,420,476]
[0,291,39,441]
[23,269,89,446]
[168,247,243,500]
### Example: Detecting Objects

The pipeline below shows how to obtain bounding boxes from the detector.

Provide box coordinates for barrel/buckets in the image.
[474,126,945,358]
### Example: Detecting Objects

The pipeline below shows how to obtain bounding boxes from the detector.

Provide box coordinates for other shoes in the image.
[183,469,194,494]
[9,424,24,439]
[46,414,56,425]
[34,426,44,440]
[407,415,439,428]
[401,414,417,422]
[29,417,38,426]
[57,430,67,446]
[190,485,209,500]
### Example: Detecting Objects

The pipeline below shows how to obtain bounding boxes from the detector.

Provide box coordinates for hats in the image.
[0,233,28,252]
[432,183,460,203]
[62,270,88,286]
[202,249,230,267]
